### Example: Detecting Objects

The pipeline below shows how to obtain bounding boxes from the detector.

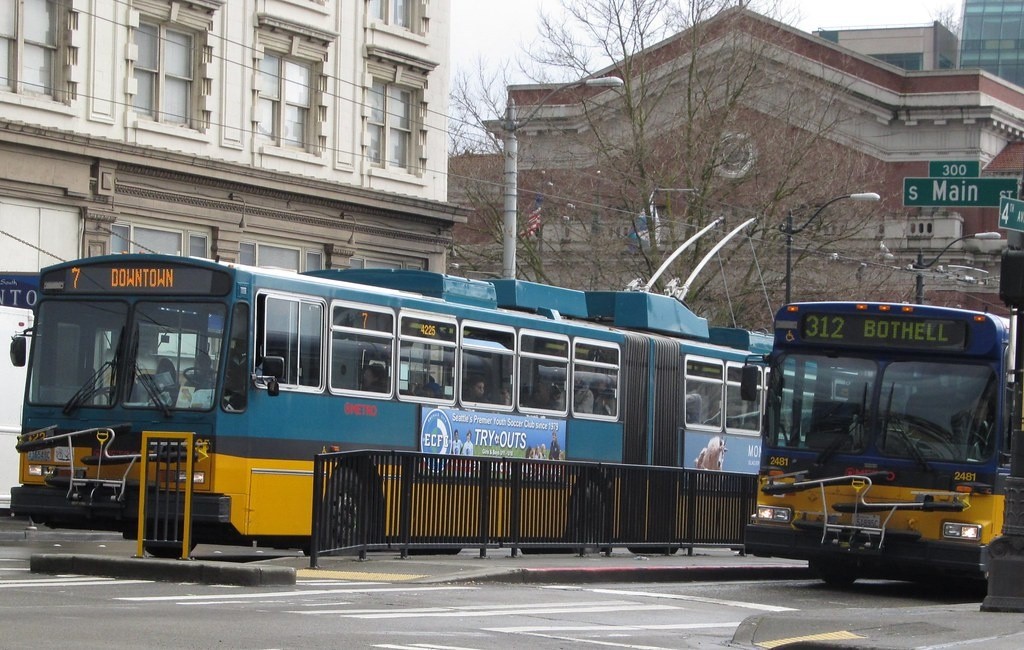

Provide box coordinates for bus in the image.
[7,249,913,563]
[734,297,1011,592]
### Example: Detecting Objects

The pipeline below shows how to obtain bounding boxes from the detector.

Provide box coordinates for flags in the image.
[628,196,661,247]
[520,194,543,238]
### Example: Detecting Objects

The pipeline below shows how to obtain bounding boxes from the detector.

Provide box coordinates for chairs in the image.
[904,392,954,441]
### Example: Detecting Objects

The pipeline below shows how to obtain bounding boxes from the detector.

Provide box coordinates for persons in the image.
[408,374,443,399]
[364,362,387,393]
[970,397,991,427]
[186,352,211,389]
[463,380,511,404]
[452,430,473,455]
[526,431,560,460]
[156,356,176,385]
[556,375,594,412]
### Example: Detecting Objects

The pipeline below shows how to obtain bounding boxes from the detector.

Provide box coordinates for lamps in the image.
[438,228,456,258]
[230,193,246,229]
[341,212,357,245]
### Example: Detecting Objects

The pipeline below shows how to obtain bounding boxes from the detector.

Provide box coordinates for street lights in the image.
[779,191,883,304]
[913,227,1005,305]
[501,75,624,275]
[980,247,1024,616]
